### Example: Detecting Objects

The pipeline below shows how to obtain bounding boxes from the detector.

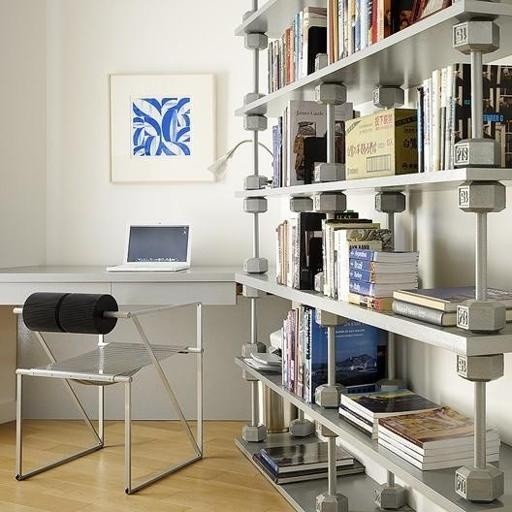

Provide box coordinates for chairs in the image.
[12,292,204,496]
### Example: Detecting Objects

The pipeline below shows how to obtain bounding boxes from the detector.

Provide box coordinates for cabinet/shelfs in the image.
[234,0,512,512]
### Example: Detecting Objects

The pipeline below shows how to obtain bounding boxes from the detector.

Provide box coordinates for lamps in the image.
[208,140,274,176]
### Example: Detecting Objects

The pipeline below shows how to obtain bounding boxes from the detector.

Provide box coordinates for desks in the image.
[0,265,237,306]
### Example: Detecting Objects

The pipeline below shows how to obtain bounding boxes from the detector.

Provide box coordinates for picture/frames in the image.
[110,75,218,183]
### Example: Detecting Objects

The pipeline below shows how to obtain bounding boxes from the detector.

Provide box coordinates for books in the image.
[265,0,447,93]
[251,305,502,470]
[273,211,512,327]
[271,64,512,187]
[252,442,366,485]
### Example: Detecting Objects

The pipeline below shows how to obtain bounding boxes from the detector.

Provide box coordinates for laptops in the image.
[107,221,192,272]
